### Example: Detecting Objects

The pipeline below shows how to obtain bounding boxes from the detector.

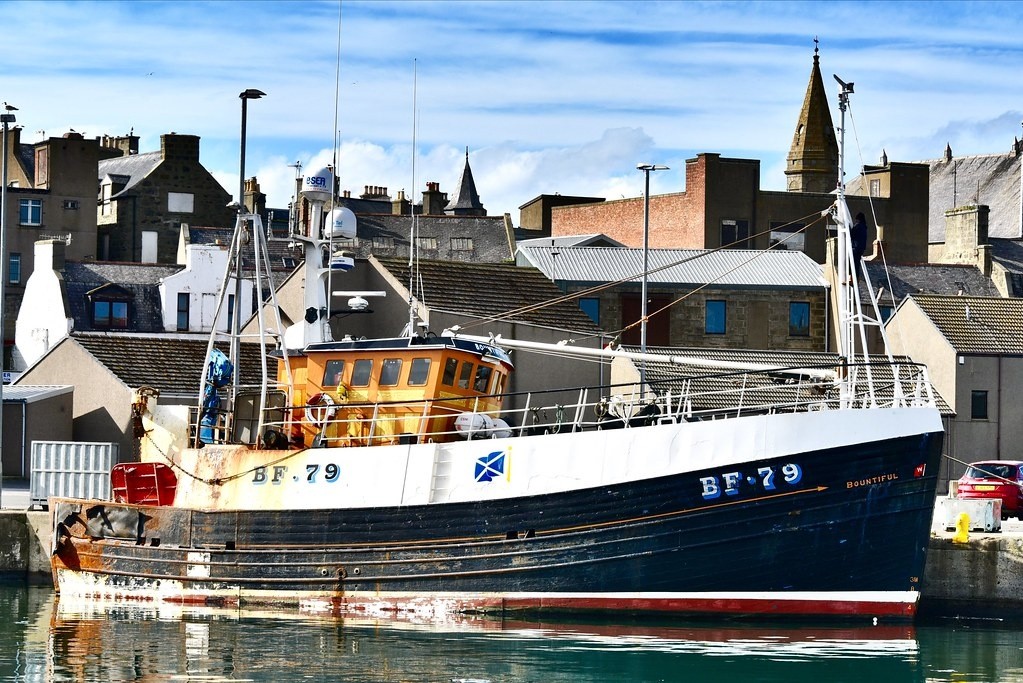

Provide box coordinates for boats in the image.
[6,74,947,619]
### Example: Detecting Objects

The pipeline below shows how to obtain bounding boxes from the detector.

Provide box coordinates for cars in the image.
[957,460,1023,521]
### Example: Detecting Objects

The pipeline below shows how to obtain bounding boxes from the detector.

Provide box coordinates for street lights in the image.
[635,162,671,411]
[233,87,267,393]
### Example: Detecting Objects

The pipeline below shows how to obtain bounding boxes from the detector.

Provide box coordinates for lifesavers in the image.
[304,391,337,426]
